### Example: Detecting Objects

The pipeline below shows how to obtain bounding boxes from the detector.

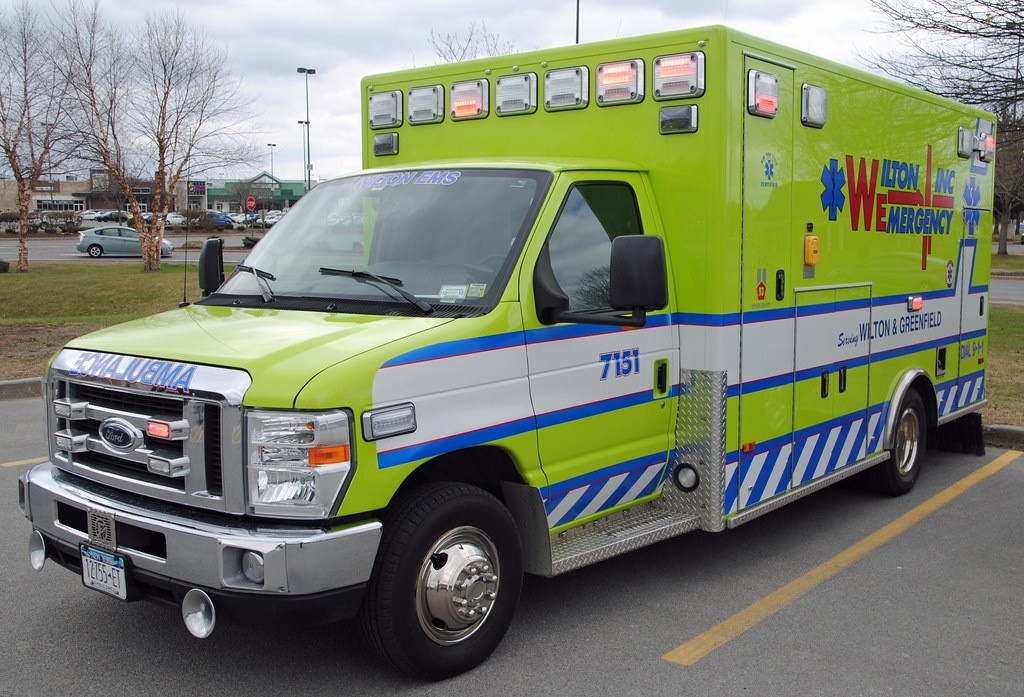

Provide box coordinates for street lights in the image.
[298,120,310,193]
[297,67,316,189]
[267,143,276,209]
[41,122,54,211]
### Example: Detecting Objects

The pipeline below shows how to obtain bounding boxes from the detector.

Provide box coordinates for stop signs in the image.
[246,196,256,211]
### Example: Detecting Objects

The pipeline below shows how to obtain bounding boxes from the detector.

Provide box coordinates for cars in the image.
[75,225,174,258]
[23,207,291,231]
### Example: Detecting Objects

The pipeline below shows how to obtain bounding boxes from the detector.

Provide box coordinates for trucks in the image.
[17,24,998,682]
